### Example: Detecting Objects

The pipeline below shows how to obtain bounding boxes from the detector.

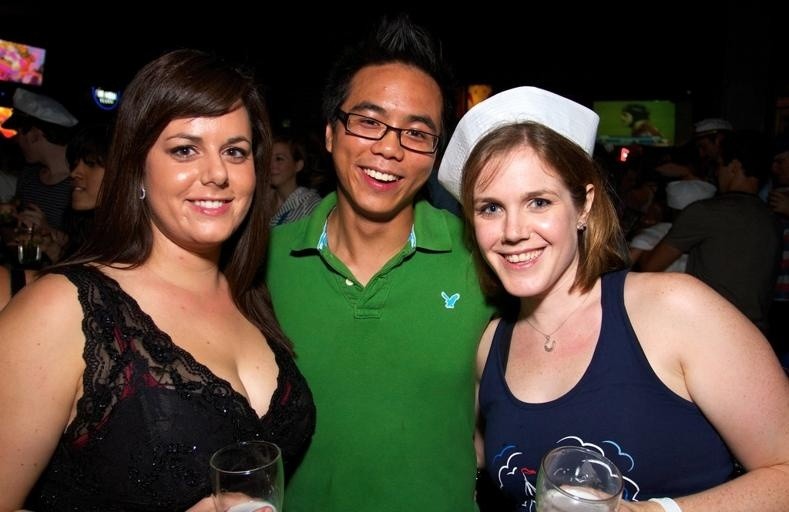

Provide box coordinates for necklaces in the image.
[525,291,589,352]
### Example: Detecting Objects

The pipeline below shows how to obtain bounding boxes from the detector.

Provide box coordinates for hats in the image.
[683,117,733,134]
[666,181,716,210]
[438,87,600,205]
[13,88,80,128]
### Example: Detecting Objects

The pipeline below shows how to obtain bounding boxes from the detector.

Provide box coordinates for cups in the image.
[533,446,624,511]
[209,438,287,512]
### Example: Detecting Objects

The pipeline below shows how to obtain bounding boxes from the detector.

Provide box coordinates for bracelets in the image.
[647,497,681,512]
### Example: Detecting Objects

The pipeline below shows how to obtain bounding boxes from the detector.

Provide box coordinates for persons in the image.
[0,90,116,309]
[264,15,510,511]
[593,116,788,336]
[268,135,470,228]
[438,84,786,512]
[1,46,316,509]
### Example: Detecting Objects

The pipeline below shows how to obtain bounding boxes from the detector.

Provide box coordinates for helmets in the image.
[622,105,649,121]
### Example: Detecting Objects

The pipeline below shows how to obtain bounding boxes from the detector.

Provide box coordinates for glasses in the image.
[333,107,442,154]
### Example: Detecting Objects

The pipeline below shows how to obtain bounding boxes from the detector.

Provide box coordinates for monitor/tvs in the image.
[591,99,677,149]
[0,38,47,88]
